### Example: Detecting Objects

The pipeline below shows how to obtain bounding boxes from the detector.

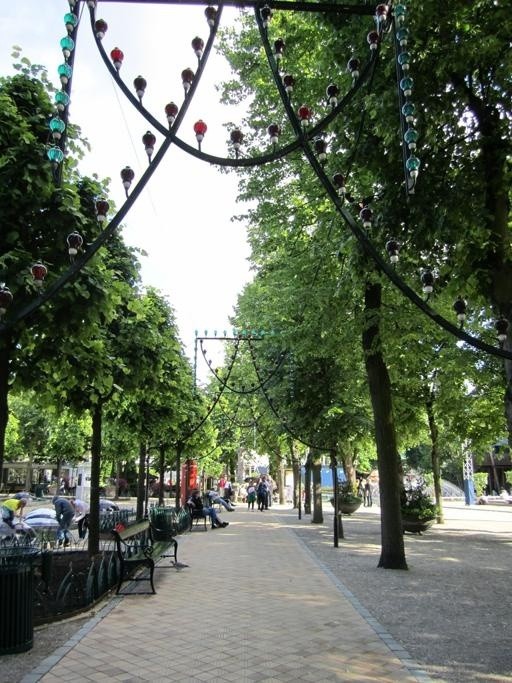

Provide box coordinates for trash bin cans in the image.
[0,547,40,656]
[149,507,172,541]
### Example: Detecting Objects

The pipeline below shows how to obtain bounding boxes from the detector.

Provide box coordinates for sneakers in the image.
[211,522,228,529]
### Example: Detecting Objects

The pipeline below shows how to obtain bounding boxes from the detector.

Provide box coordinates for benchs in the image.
[111,519,178,594]
[207,493,221,513]
[191,495,216,532]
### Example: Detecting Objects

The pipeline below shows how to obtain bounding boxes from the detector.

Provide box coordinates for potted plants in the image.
[402,489,441,538]
[331,483,362,517]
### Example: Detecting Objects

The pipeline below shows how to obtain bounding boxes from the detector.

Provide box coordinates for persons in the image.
[182,470,276,533]
[354,478,374,508]
[50,494,74,545]
[1,497,29,526]
[114,476,131,496]
[69,497,92,542]
[150,477,161,494]
